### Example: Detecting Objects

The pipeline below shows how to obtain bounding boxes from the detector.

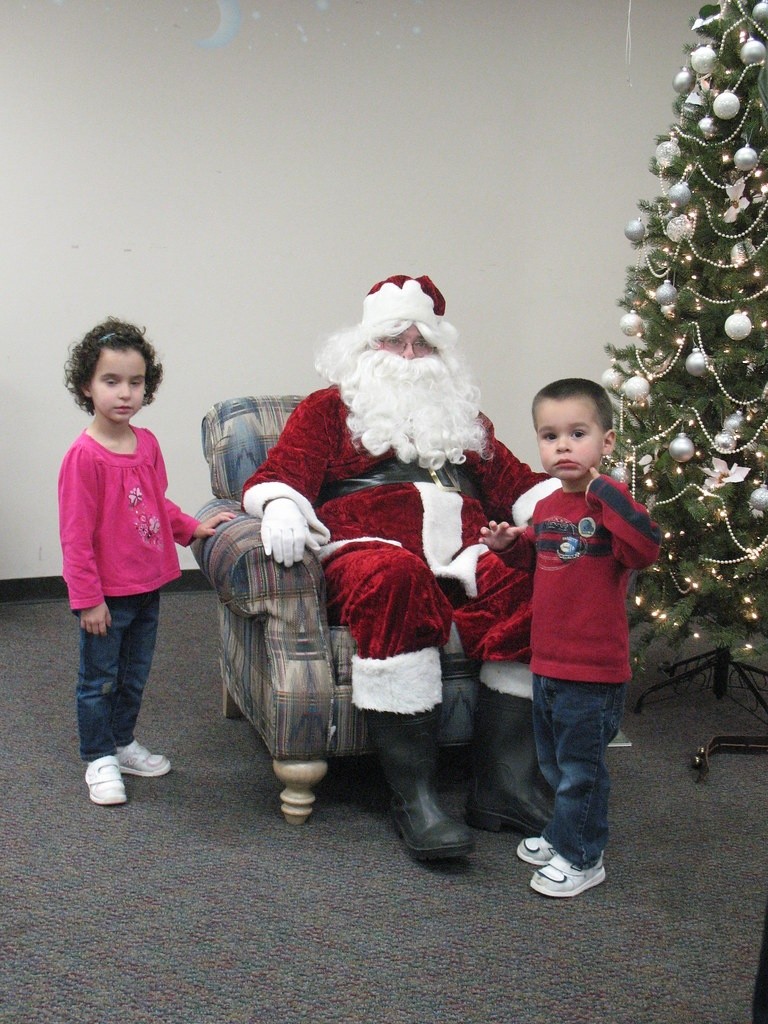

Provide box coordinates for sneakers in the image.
[529,850,606,897]
[116,740,171,777]
[85,756,127,805]
[516,835,557,865]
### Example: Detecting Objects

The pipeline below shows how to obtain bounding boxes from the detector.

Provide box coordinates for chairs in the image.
[188,394,479,826]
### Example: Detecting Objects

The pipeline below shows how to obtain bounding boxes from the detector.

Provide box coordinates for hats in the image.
[361,275,445,339]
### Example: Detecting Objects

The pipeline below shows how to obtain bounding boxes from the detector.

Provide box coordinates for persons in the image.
[59,318,236,805]
[242,275,556,859]
[479,378,661,898]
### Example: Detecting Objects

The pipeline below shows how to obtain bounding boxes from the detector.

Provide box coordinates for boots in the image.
[463,661,555,838]
[350,646,474,861]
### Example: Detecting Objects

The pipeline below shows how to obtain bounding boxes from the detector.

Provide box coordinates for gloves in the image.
[261,497,328,567]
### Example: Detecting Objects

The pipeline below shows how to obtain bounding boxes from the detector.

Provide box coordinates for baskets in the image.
[324,456,481,496]
